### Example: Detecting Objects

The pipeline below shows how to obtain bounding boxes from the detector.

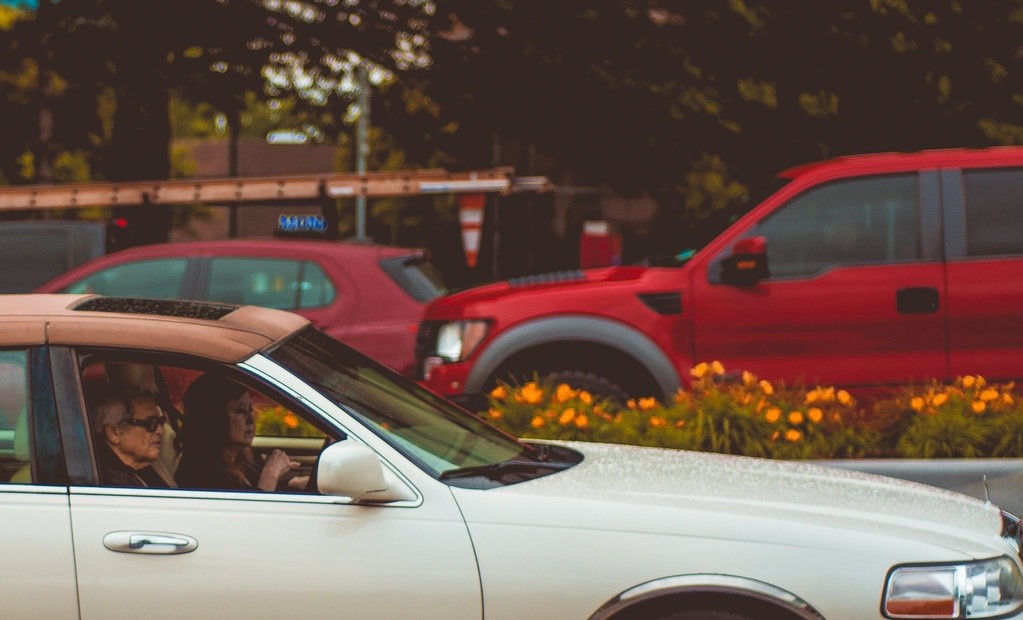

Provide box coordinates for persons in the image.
[86,387,168,489]
[173,372,310,491]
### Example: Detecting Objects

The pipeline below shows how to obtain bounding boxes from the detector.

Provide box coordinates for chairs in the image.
[15,401,184,496]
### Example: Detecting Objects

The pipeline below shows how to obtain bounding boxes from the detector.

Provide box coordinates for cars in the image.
[1,293,1021,620]
[0,238,443,370]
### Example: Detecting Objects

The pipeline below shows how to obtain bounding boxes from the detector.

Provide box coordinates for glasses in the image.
[127,414,167,432]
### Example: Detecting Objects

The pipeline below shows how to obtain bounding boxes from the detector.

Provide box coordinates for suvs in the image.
[417,143,1023,415]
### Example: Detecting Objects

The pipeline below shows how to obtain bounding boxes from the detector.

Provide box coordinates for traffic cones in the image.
[454,194,486,268]
